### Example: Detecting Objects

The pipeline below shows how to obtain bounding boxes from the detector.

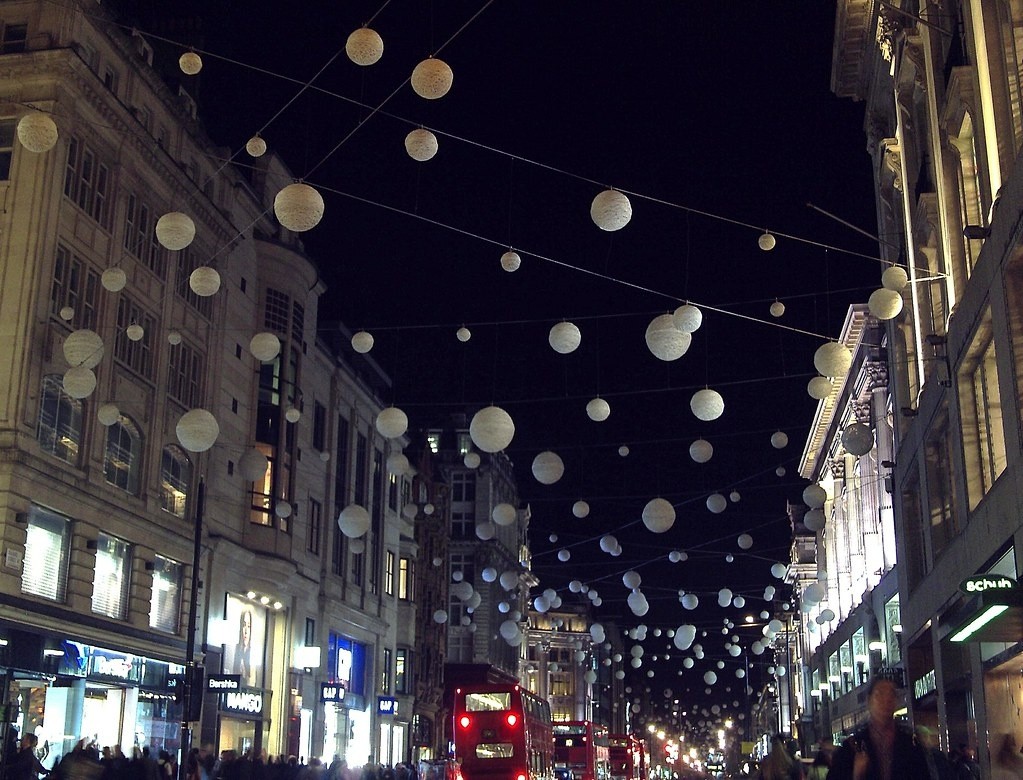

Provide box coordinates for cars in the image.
[553,766,575,780]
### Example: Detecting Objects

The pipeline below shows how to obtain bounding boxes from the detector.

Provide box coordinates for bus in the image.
[551,720,640,780]
[453,685,555,780]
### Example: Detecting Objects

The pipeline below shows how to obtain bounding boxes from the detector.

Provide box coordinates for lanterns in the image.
[17,27,907,772]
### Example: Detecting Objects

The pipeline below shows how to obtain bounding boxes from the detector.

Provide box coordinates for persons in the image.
[233,610,251,687]
[0,728,451,780]
[755,680,980,780]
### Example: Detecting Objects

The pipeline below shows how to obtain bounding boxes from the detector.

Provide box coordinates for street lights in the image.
[673,706,687,757]
[648,723,655,773]
